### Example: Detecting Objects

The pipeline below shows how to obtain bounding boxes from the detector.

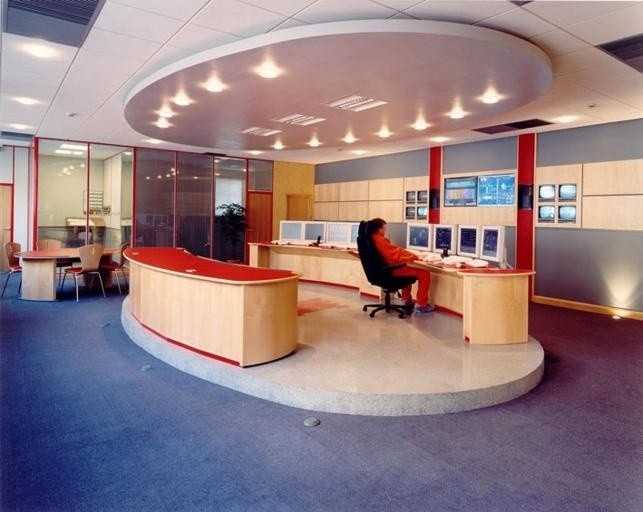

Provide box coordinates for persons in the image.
[370,217,437,314]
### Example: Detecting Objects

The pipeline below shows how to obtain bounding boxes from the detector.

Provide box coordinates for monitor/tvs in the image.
[405,190,428,221]
[535,182,578,225]
[278,220,512,270]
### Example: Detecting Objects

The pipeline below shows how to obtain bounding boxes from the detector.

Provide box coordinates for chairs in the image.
[357,219,418,318]
[1,239,130,304]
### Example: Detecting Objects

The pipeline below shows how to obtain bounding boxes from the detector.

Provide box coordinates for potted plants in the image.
[217,204,248,263]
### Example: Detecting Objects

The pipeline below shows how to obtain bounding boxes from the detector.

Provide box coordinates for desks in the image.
[123,246,303,369]
[247,242,536,346]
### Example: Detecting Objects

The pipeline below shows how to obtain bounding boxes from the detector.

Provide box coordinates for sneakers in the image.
[401,302,435,313]
